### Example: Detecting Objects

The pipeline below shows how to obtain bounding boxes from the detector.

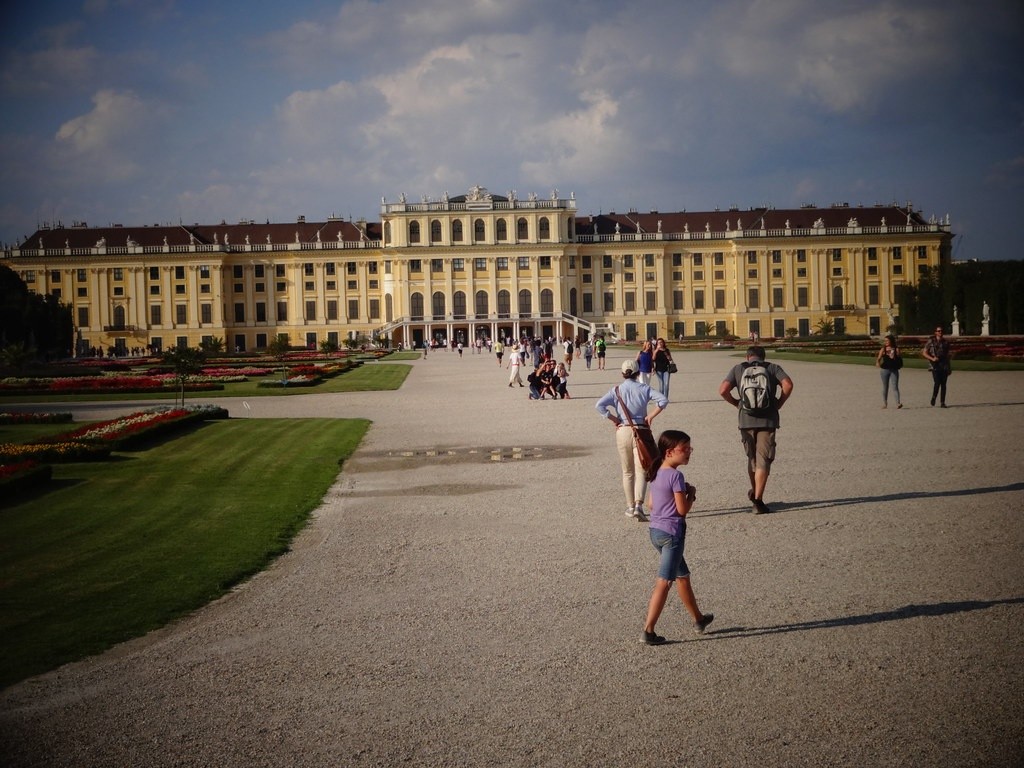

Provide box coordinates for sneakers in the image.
[640,631,666,645]
[625,505,647,522]
[693,612,714,633]
[747,489,770,513]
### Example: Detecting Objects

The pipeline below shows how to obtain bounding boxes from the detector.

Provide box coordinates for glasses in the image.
[671,446,694,452]
[936,330,943,332]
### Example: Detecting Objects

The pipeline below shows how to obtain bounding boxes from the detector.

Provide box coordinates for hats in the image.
[622,359,639,375]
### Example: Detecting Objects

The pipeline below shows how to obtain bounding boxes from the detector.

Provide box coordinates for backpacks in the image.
[567,341,573,353]
[739,361,775,416]
[598,341,606,351]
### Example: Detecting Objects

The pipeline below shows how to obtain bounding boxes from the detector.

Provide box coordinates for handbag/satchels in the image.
[667,364,677,373]
[633,429,659,470]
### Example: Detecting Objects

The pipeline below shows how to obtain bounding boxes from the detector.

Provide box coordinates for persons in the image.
[126,344,174,356]
[749,329,759,342]
[398,336,606,400]
[595,359,669,522]
[635,338,672,399]
[876,335,903,409]
[639,430,714,644]
[679,332,684,344]
[720,346,793,512]
[809,328,813,336]
[236,345,240,353]
[923,326,952,408]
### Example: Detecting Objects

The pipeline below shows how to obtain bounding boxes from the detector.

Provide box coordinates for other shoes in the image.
[509,383,514,388]
[553,393,557,400]
[598,367,605,370]
[882,401,888,408]
[528,393,532,399]
[896,400,902,409]
[940,403,948,408]
[568,367,571,371]
[586,367,590,371]
[931,397,936,406]
[519,383,524,387]
[540,395,545,400]
